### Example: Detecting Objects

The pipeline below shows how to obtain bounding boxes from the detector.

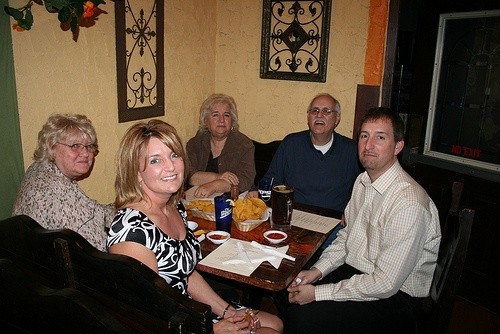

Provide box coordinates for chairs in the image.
[424,182,475,334]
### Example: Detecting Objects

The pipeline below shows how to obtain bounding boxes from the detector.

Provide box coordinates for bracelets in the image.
[223,304,230,319]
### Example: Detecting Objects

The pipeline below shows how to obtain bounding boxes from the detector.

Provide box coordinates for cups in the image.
[270,185,296,230]
[214,195,233,233]
[258,179,274,207]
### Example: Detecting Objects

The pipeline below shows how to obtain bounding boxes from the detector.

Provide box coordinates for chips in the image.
[183,200,215,212]
[232,196,267,221]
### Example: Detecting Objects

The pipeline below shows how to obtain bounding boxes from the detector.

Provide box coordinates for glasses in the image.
[57,142,98,153]
[308,108,338,115]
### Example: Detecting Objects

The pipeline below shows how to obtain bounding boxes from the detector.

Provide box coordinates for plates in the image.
[206,231,231,244]
[263,231,287,244]
[187,221,198,231]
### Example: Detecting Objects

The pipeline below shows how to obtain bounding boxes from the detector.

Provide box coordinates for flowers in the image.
[3,0,107,34]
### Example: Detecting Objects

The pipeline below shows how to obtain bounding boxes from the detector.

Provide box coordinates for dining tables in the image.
[186,203,341,291]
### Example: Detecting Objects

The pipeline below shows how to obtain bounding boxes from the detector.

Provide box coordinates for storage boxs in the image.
[231,209,269,232]
[190,207,215,221]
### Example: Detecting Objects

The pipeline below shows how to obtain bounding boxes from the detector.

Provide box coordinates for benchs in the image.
[0,140,284,334]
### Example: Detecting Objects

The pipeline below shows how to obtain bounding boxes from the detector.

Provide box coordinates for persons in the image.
[183,93,256,198]
[106,119,284,334]
[287,107,442,334]
[248,93,365,270]
[12,112,117,253]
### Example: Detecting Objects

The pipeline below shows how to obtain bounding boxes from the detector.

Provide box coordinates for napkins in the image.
[223,245,289,270]
